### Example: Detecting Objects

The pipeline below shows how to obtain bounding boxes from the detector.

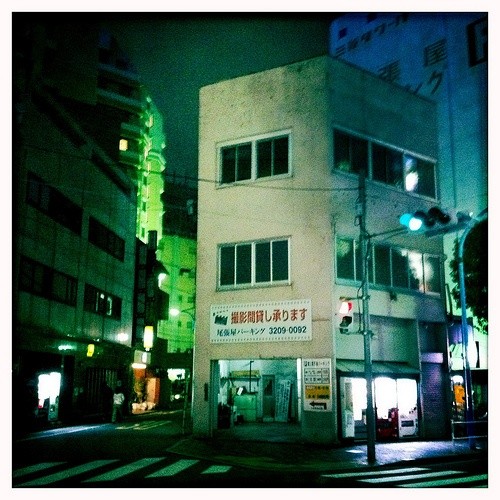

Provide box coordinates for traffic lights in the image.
[408,206,452,232]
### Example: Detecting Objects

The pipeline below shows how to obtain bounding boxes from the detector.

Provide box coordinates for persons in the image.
[111,386,126,424]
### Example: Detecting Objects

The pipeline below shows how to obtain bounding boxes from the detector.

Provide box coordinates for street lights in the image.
[339,294,376,465]
